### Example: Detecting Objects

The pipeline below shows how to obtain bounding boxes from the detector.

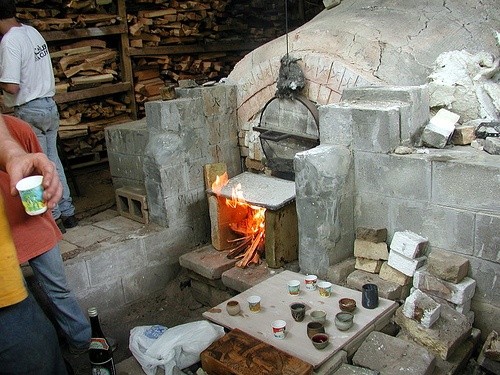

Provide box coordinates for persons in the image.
[0,0,79,234]
[0,190,69,375]
[0,113,117,355]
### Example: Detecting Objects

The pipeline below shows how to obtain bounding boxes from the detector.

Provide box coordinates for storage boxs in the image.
[200,328,313,375]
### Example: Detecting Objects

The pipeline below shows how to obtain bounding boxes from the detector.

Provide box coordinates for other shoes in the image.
[61,215,78,228]
[55,218,66,234]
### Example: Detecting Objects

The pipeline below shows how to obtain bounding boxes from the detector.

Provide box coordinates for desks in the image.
[202,269,397,372]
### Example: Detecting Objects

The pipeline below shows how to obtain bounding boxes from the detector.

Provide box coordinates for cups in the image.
[16,175,48,215]
[339,298,356,312]
[304,274,317,291]
[310,310,326,324]
[287,279,300,296]
[271,320,287,340]
[311,333,328,349]
[290,303,306,322]
[318,281,332,299]
[248,296,261,314]
[307,322,325,339]
[335,312,353,331]
[226,301,240,315]
[362,284,378,309]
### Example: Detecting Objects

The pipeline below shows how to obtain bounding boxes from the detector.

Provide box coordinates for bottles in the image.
[88,306,116,375]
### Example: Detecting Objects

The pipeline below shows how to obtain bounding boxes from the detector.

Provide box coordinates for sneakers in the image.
[68,337,118,357]
